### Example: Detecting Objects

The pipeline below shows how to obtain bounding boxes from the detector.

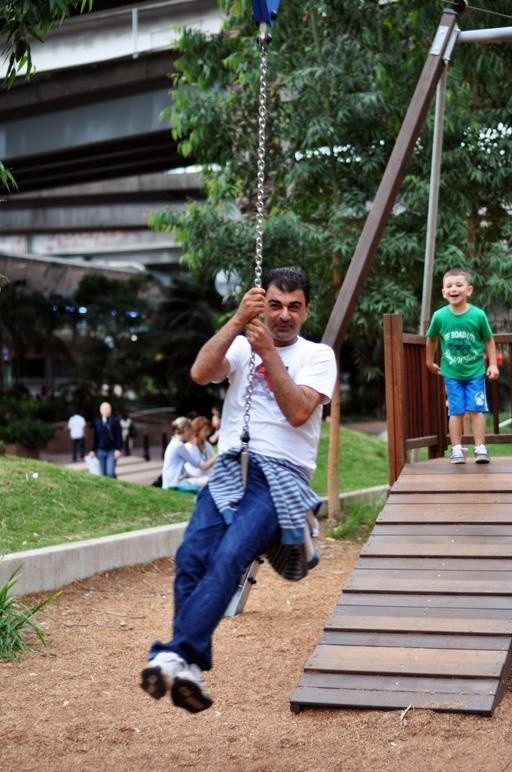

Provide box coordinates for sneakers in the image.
[450,444,467,465]
[170,665,213,715]
[474,443,490,464]
[140,652,185,698]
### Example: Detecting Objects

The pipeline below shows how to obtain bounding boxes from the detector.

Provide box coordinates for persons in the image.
[426,269,500,463]
[66,380,221,493]
[141,272,337,714]
